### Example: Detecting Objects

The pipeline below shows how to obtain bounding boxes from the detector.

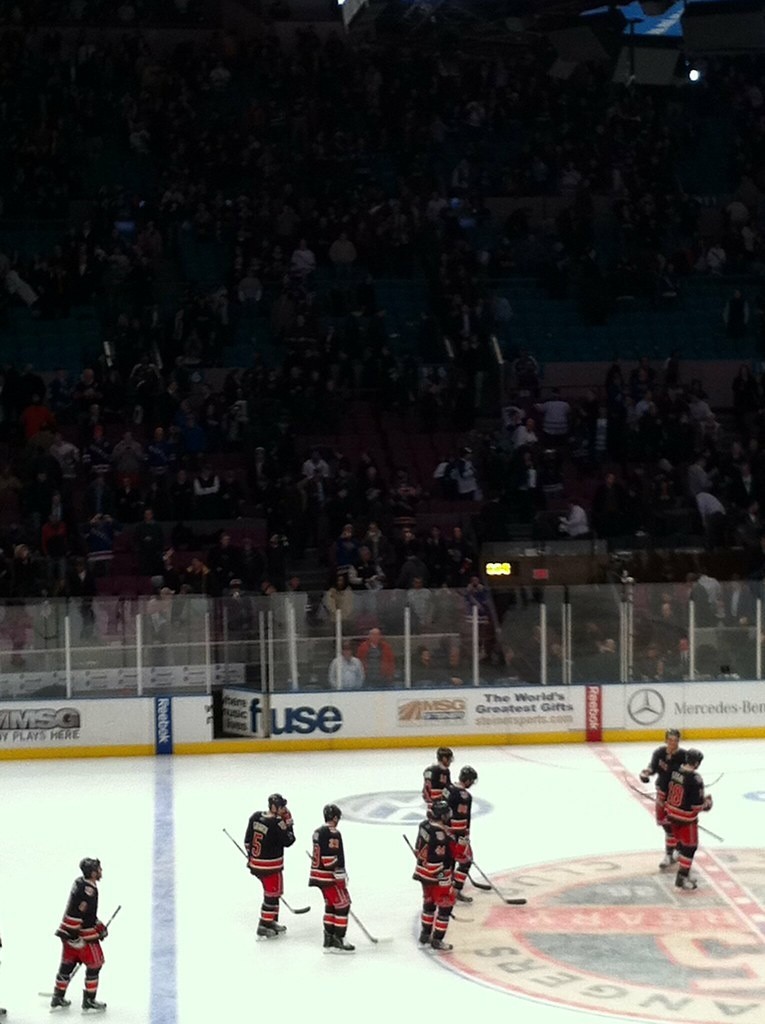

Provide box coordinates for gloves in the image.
[702,795,713,811]
[639,769,651,783]
[335,874,346,891]
[96,921,107,941]
[67,937,85,949]
[281,807,293,825]
[456,836,468,859]
[439,881,454,897]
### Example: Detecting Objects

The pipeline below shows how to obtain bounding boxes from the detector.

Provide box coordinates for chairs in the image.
[0,195,765,599]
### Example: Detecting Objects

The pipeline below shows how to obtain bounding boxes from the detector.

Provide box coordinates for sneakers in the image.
[673,875,697,893]
[255,920,281,942]
[453,891,473,907]
[417,931,431,949]
[659,854,678,872]
[50,987,71,1010]
[271,922,287,934]
[329,935,355,954]
[428,932,455,955]
[82,990,107,1014]
[322,931,334,953]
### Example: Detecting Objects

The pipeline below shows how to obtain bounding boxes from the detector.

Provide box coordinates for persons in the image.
[640,729,689,871]
[411,800,466,954]
[665,749,712,892]
[0,22,765,690]
[243,794,295,942]
[442,765,478,905]
[308,804,357,954]
[49,858,108,1015]
[421,747,454,819]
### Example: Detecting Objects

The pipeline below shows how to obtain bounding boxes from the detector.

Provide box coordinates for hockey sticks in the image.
[463,849,528,905]
[467,871,492,891]
[641,772,724,796]
[222,828,312,915]
[404,834,476,924]
[305,850,395,945]
[621,771,724,843]
[38,904,122,999]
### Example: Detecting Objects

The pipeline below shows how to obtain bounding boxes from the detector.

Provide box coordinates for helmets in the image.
[683,749,703,763]
[431,801,449,816]
[323,804,339,820]
[461,766,478,781]
[79,858,100,878]
[665,728,680,739]
[268,794,286,807]
[437,747,452,760]
